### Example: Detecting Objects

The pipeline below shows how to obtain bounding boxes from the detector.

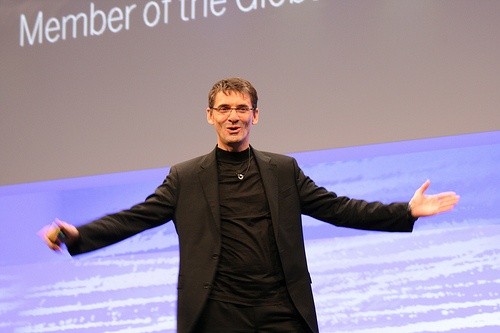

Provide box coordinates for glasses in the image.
[210,105,254,113]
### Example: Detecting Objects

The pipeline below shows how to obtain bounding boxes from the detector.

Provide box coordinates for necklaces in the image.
[227,146,250,179]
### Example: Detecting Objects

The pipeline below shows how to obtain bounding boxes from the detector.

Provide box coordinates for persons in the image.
[39,79,459,333]
